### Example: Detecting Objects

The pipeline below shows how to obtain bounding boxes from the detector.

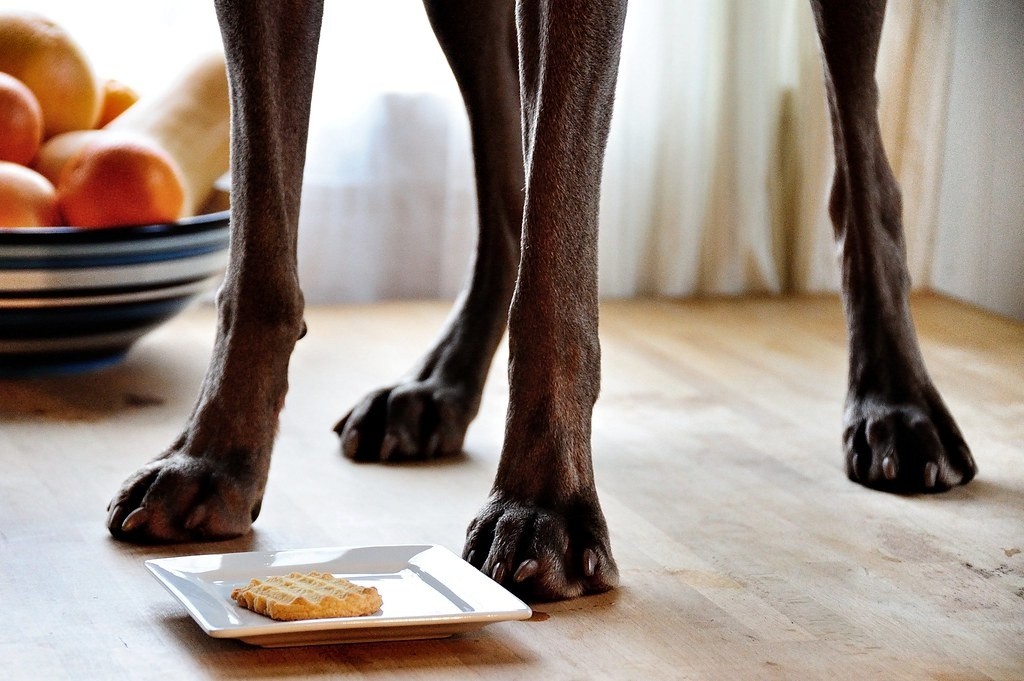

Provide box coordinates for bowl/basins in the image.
[0,185,232,378]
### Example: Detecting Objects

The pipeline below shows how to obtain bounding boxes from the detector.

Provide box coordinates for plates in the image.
[144,542,533,648]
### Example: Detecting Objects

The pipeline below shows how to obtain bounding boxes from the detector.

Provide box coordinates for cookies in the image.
[231,572,384,621]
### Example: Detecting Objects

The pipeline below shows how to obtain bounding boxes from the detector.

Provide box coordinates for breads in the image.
[98,51,230,204]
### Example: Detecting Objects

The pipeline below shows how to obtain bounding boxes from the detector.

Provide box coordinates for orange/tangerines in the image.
[0,5,180,231]
